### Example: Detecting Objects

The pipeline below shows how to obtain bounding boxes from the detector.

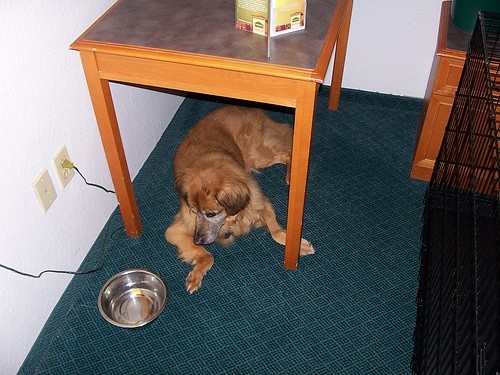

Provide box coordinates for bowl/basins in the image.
[97,268,169,328]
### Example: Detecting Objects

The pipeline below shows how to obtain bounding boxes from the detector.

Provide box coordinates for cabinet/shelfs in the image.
[410,0,500,198]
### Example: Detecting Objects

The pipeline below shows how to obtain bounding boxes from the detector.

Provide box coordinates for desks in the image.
[70,0,355,270]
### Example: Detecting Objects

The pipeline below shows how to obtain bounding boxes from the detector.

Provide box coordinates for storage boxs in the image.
[235,0,307,37]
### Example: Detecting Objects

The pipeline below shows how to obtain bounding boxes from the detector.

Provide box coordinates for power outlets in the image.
[34,169,57,213]
[53,146,75,188]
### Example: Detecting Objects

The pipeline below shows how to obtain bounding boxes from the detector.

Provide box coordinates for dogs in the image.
[164,105,316,295]
